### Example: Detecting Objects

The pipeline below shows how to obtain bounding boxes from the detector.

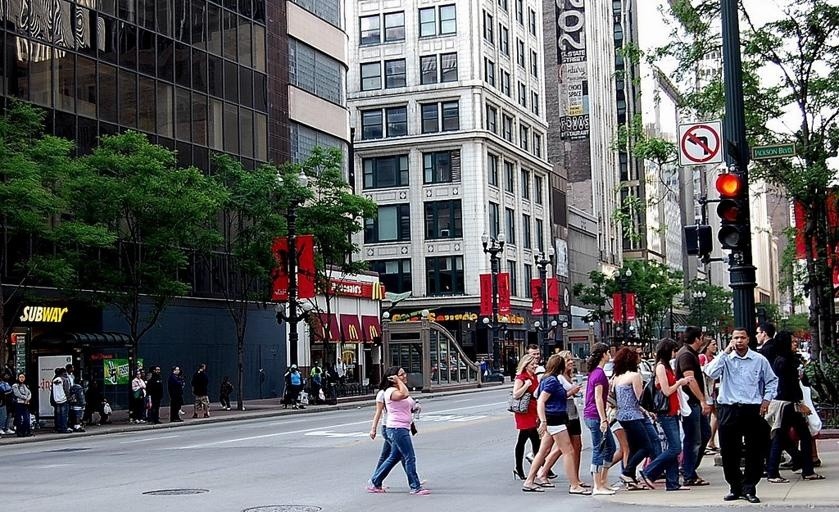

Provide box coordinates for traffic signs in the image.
[751,144,795,159]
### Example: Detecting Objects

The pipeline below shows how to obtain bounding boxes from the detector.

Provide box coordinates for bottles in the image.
[414,398,420,419]
[574,388,584,407]
[706,396,713,405]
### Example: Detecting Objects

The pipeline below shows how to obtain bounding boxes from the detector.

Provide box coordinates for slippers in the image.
[704,445,721,455]
[513,452,710,495]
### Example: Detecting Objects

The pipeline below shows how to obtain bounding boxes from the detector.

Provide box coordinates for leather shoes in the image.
[723,493,760,503]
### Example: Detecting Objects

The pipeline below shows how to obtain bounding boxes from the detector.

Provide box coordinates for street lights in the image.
[481,233,505,378]
[694,291,707,332]
[531,247,568,372]
[271,170,315,367]
[613,268,632,341]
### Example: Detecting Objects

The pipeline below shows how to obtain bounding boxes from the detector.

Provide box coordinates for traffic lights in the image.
[699,225,714,255]
[716,173,744,249]
[684,224,699,255]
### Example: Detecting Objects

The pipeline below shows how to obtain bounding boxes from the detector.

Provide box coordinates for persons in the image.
[219,376,234,410]
[511,324,826,503]
[1,359,212,437]
[310,361,324,403]
[283,363,306,409]
[325,361,337,405]
[334,357,346,384]
[480,357,487,383]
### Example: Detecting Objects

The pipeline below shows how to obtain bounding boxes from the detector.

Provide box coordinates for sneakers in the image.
[766,458,826,483]
[0,429,15,435]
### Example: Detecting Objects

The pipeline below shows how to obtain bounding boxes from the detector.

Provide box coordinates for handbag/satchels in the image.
[638,380,669,414]
[507,391,531,413]
[411,422,417,436]
[606,390,617,407]
[133,389,143,399]
